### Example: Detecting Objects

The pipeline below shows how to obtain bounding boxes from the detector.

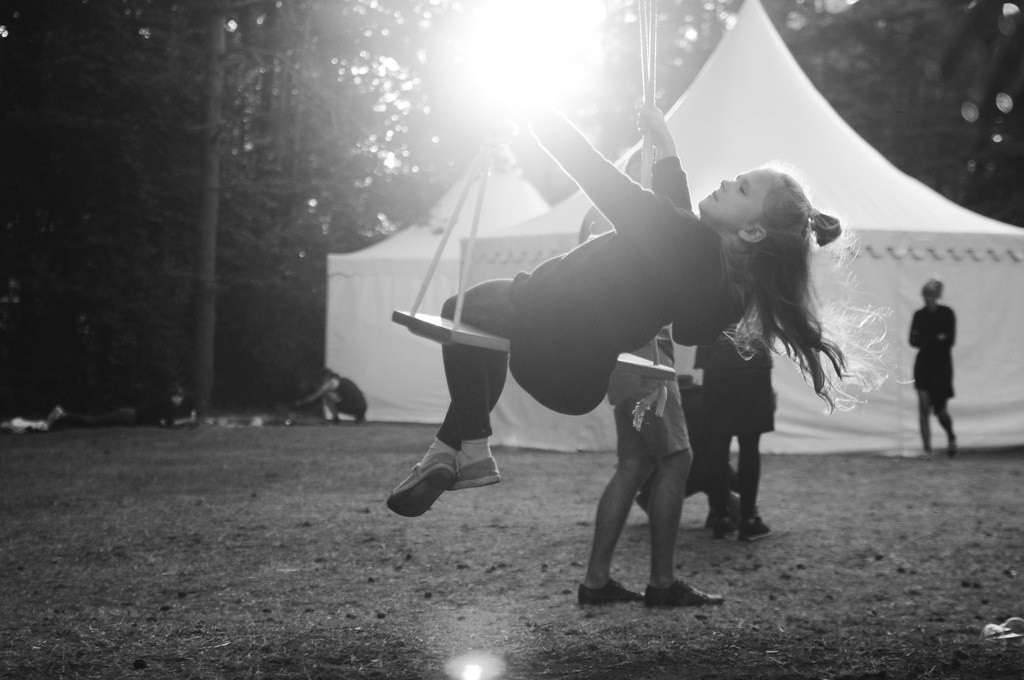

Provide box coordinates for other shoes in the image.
[579,580,644,606]
[646,581,723,605]
[714,520,737,538]
[446,456,501,490]
[387,455,456,517]
[741,517,771,540]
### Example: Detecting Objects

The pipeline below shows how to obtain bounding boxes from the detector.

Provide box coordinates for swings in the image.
[390,0,686,389]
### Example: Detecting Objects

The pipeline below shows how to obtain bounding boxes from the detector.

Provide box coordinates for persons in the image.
[692,317,782,540]
[908,279,958,461]
[294,367,367,423]
[384,87,842,518]
[45,388,196,432]
[573,148,725,607]
[634,372,737,529]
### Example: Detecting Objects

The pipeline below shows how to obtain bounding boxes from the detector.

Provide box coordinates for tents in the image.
[326,124,550,423]
[462,0,1024,454]
